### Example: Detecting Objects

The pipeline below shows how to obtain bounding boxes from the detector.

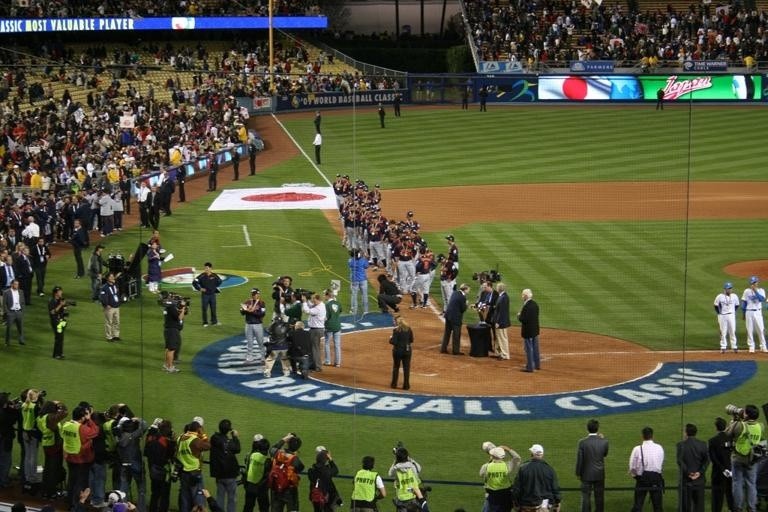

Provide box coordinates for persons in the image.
[0,0,768,512]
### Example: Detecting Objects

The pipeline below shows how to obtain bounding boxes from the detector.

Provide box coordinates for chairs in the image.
[247,0,367,98]
[1,0,246,202]
[462,0,768,71]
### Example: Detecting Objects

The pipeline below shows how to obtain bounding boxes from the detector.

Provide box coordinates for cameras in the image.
[193,416,203,428]
[148,418,163,432]
[254,434,263,442]
[407,488,414,492]
[725,404,744,419]
[56,297,76,309]
[8,397,20,405]
[38,390,47,399]
[290,432,296,438]
[316,446,329,460]
[481,441,497,455]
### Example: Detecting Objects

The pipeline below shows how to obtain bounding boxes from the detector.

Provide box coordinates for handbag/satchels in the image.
[748,441,767,466]
[641,471,664,492]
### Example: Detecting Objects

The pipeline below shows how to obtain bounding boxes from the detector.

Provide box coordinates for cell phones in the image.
[196,491,204,495]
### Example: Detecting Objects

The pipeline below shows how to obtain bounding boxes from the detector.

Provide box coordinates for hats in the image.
[749,276,758,284]
[436,254,445,263]
[249,287,259,294]
[489,447,505,459]
[335,174,381,220]
[529,444,544,455]
[445,235,454,242]
[724,283,732,289]
[401,210,426,253]
